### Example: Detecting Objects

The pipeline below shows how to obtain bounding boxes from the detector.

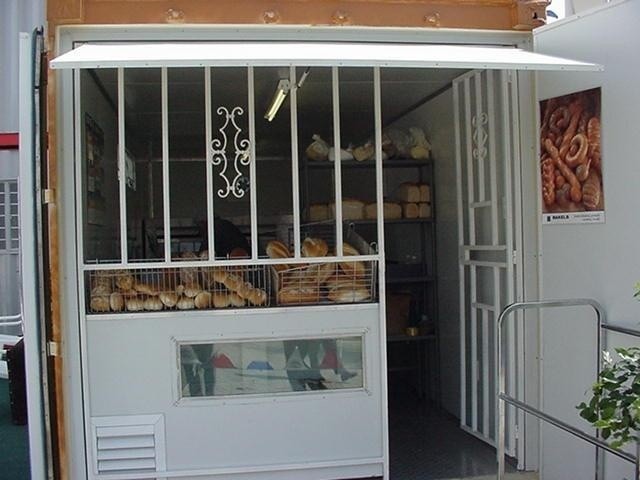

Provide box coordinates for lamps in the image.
[263,79,290,122]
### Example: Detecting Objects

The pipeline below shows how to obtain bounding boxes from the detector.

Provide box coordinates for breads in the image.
[267,237,371,303]
[310,182,431,221]
[91,251,267,311]
[307,127,429,162]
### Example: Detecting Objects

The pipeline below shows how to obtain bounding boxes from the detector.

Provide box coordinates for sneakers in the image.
[340,370,359,382]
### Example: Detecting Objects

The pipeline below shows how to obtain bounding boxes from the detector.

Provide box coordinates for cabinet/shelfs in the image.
[303,157,441,405]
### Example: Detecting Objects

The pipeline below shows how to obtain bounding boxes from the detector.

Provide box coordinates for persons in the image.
[283,340,357,391]
[193,208,251,260]
[181,344,216,396]
